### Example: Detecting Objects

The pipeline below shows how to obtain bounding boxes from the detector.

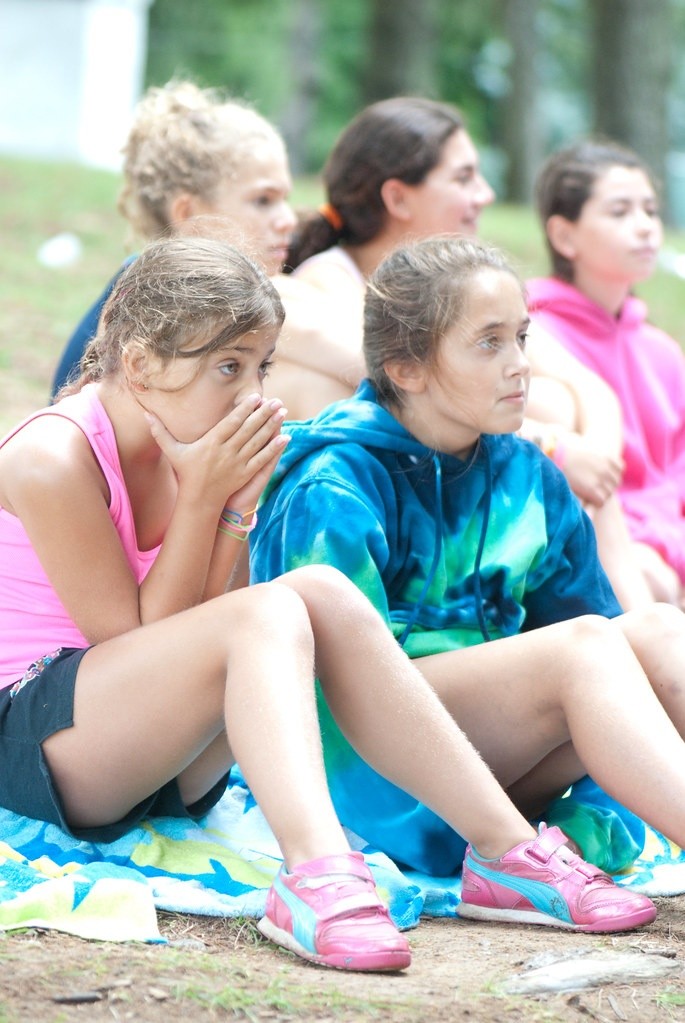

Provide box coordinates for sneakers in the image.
[455,822,657,934]
[258,852,412,970]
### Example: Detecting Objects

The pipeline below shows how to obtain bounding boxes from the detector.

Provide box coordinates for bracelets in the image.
[216,503,258,542]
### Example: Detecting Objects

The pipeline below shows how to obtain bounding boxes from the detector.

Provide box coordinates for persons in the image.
[521,138,685,587]
[48,74,372,422]
[244,235,685,879]
[264,95,685,613]
[0,233,657,973]
